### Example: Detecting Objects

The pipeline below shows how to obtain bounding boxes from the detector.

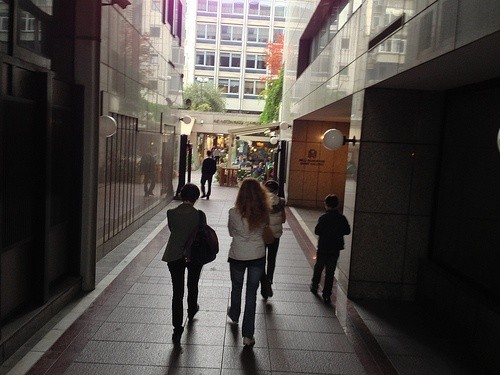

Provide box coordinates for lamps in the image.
[321,128,356,155]
[270,135,282,146]
[100,116,117,139]
[180,117,192,124]
[282,122,292,131]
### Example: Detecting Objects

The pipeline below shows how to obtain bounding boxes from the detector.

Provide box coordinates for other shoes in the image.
[226,307,239,324]
[310,285,333,305]
[261,285,274,300]
[170,345,184,358]
[242,335,257,346]
[200,194,209,200]
[187,304,199,323]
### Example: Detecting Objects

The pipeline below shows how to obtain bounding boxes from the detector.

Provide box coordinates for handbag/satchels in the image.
[264,228,275,244]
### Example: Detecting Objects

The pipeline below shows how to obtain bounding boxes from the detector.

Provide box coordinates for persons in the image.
[141,147,157,196]
[234,150,264,177]
[211,146,224,164]
[200,150,216,199]
[309,194,351,303]
[162,183,207,337]
[226,177,272,346]
[256,180,287,299]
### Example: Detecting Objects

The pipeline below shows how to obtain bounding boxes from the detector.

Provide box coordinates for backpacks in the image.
[185,224,219,267]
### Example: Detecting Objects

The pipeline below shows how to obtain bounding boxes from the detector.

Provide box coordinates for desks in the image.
[220,166,240,188]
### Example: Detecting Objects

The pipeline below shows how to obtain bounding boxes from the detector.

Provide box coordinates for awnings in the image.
[228,122,279,137]
